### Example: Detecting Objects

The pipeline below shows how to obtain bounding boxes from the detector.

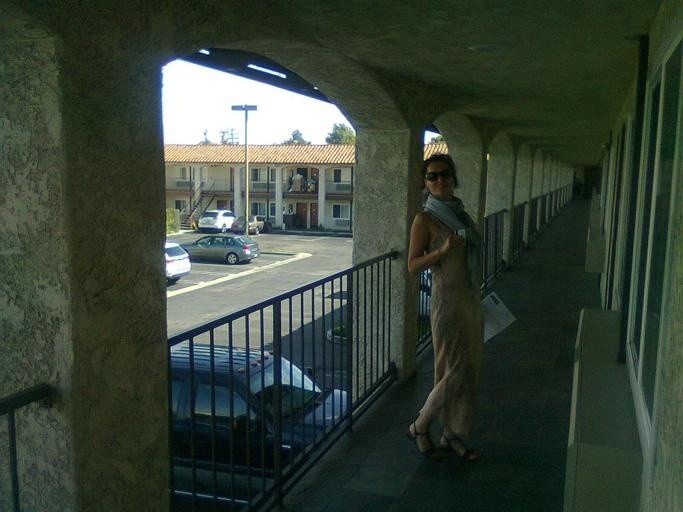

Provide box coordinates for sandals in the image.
[438,433,481,465]
[406,419,441,462]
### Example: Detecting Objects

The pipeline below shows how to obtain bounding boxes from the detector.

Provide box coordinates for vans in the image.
[165,242,192,285]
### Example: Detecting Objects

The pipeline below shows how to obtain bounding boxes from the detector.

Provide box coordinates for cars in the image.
[229,213,273,235]
[418,268,430,320]
[177,233,261,266]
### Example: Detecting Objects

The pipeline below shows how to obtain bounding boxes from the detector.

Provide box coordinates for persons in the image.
[404,151,485,462]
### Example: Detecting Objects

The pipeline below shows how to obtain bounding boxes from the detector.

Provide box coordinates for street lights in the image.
[230,103,257,236]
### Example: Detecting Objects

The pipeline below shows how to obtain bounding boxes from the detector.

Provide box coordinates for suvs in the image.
[168,342,350,509]
[196,208,236,233]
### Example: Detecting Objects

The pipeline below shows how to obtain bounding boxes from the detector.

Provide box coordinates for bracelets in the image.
[438,247,442,257]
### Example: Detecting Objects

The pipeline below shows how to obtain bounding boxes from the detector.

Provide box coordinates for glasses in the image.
[424,169,454,181]
